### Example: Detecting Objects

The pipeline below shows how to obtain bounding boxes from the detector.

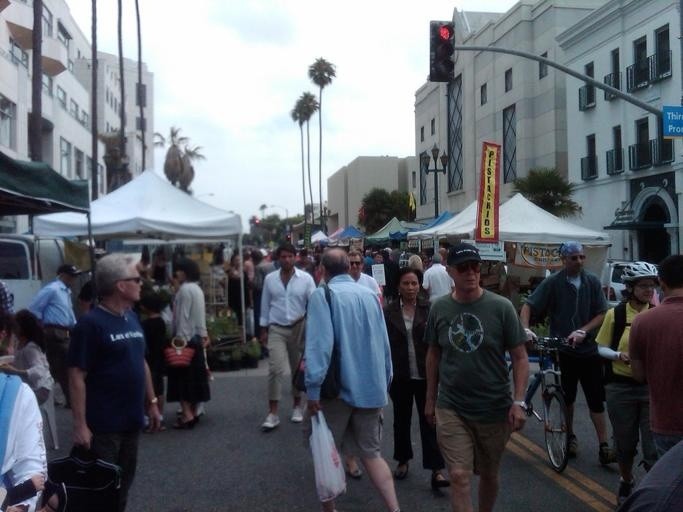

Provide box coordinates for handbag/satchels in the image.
[41,442,123,512]
[163,336,195,367]
[293,340,341,402]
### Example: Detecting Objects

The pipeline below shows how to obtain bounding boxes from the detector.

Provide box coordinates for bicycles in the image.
[503,336,575,472]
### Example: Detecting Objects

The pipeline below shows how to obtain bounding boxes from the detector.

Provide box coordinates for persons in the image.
[420,242,530,512]
[338,436,365,479]
[518,238,615,469]
[0,370,49,512]
[0,472,66,511]
[593,257,660,512]
[302,246,402,512]
[616,436,682,511]
[627,252,683,465]
[381,265,452,490]
[66,249,161,511]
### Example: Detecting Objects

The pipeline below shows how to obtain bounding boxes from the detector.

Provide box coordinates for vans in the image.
[598,262,628,308]
[0,234,74,314]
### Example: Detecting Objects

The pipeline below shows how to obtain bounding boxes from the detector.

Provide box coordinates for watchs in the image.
[512,399,528,412]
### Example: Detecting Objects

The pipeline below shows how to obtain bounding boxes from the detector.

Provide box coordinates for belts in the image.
[270,315,306,330]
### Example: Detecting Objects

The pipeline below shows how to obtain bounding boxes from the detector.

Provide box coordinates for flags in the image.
[409,192,416,212]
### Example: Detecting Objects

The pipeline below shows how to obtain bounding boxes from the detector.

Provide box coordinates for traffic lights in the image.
[254,216,261,225]
[430,19,456,84]
[286,232,291,241]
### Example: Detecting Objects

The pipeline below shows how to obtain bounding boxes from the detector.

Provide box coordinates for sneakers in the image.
[291,406,304,423]
[565,435,578,454]
[599,448,619,465]
[617,476,635,505]
[260,413,280,429]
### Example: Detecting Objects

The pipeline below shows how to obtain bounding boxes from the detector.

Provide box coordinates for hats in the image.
[446,244,483,265]
[560,242,583,257]
[55,264,83,275]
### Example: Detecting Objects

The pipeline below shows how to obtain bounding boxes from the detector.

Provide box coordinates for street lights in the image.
[422,144,451,218]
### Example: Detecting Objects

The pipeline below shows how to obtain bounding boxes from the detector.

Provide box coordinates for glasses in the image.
[637,284,657,289]
[450,263,481,274]
[114,276,141,284]
[570,255,586,260]
[350,260,362,267]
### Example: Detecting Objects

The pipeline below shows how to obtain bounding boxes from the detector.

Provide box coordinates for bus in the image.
[105,237,234,281]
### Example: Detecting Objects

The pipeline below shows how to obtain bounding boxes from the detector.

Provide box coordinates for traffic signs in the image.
[663,106,683,138]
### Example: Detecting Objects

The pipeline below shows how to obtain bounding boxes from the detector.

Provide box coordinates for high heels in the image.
[171,408,203,430]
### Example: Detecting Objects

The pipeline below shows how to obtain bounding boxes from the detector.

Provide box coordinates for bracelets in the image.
[574,328,587,334]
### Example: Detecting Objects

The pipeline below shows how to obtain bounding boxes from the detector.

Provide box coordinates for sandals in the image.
[393,462,409,479]
[431,473,451,488]
[345,465,363,479]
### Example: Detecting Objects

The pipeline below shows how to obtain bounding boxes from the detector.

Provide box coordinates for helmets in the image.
[621,261,659,285]
